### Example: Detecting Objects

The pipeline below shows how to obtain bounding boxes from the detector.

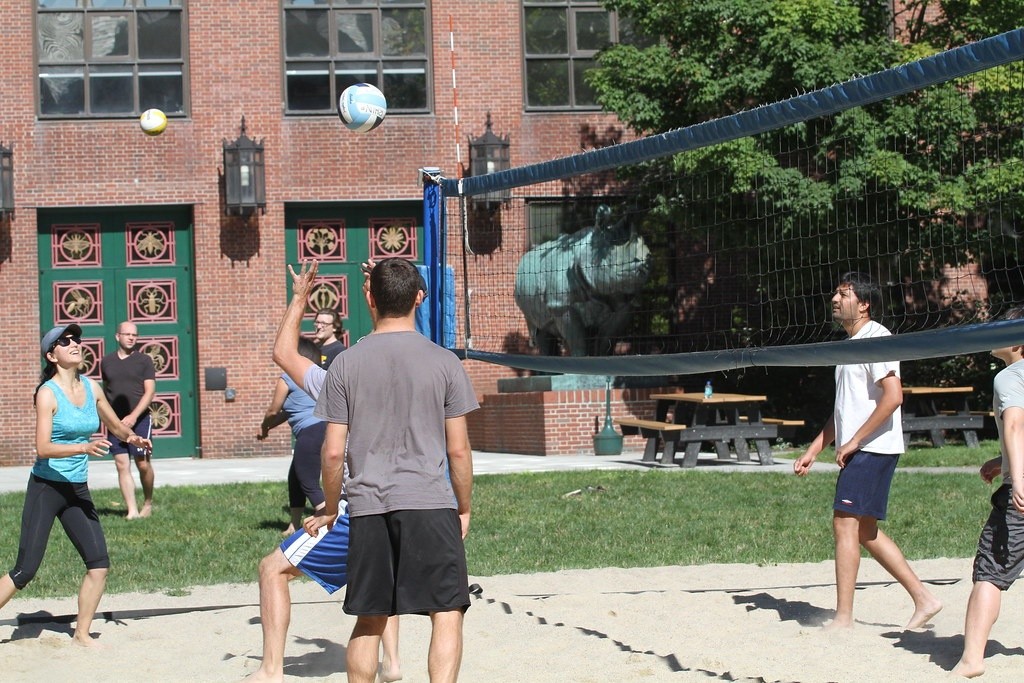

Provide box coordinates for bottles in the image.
[704,381,712,399]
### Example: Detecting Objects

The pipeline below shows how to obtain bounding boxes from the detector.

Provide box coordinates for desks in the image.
[899,385,980,452]
[651,392,768,468]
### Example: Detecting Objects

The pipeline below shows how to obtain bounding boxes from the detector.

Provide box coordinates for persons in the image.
[792,272,943,629]
[100,322,156,520]
[949,307,1024,676]
[303,256,481,683]
[0,322,152,646]
[237,256,459,682]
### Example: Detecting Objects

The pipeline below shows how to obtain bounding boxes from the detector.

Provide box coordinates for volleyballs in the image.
[140,109,167,136]
[338,83,387,133]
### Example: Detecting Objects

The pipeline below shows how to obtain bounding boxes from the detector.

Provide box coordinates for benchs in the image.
[613,416,805,473]
[940,409,997,449]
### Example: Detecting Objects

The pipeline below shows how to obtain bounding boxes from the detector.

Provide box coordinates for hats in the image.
[41,324,82,355]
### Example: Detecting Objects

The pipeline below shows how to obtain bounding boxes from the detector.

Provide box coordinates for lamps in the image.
[226,115,267,229]
[467,110,511,212]
[0,140,14,224]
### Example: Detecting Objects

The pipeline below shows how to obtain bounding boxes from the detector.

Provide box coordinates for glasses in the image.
[50,336,81,353]
[313,320,333,327]
